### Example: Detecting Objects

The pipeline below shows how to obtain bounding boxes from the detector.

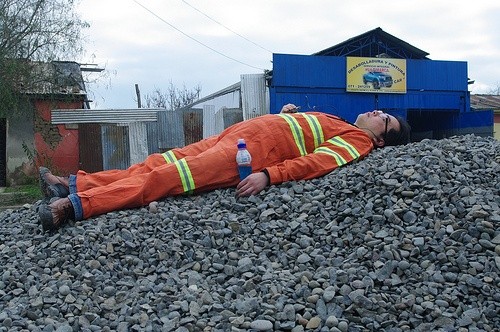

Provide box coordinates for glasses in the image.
[378,110,391,136]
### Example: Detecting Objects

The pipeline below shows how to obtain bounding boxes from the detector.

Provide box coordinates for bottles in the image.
[235,138,253,181]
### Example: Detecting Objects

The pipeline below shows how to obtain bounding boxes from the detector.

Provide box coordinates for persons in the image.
[39,104,409,231]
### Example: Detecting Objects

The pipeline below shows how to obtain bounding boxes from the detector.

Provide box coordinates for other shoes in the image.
[40,197,73,232]
[38,166,70,199]
